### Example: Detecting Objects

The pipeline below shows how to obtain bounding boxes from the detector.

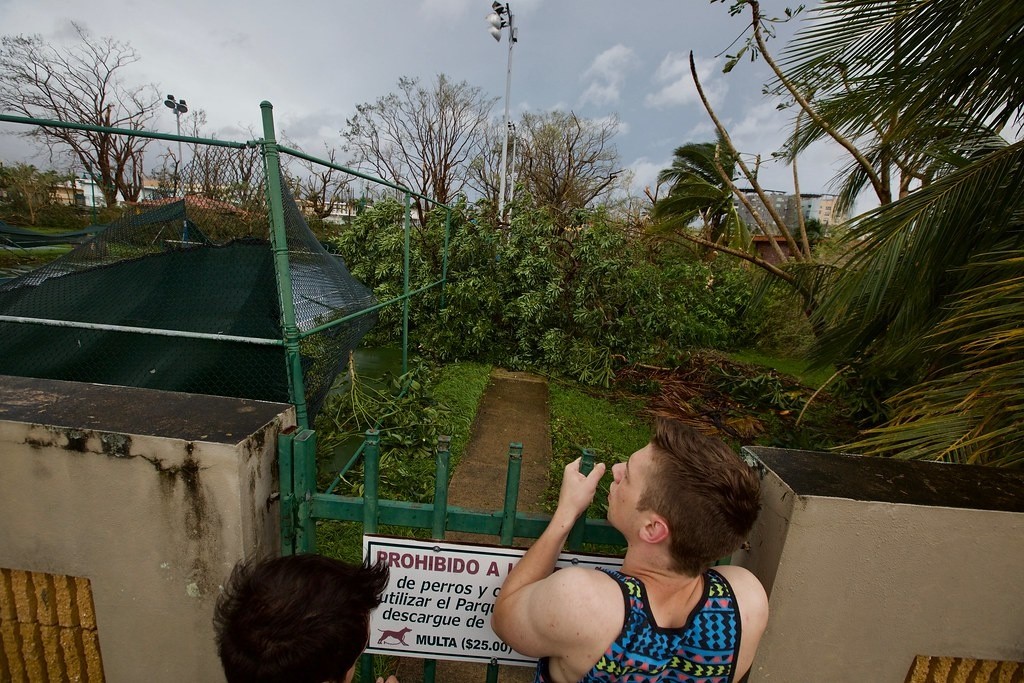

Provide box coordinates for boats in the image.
[165,240,205,248]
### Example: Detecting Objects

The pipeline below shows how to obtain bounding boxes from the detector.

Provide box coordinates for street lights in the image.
[507,121,516,229]
[164,93,189,198]
[485,2,519,232]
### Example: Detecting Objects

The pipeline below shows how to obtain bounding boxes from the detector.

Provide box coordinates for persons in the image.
[212,552,399,683]
[490,418,768,683]
